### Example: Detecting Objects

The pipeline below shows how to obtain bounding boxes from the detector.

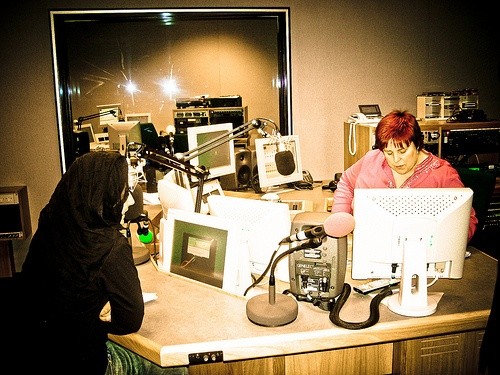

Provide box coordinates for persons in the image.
[332,110,478,244]
[1,151,144,375]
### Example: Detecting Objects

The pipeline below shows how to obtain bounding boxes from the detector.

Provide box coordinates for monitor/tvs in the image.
[351,188,473,317]
[125,113,151,123]
[187,123,236,182]
[255,135,303,187]
[107,121,142,150]
[74,123,95,143]
[157,164,292,294]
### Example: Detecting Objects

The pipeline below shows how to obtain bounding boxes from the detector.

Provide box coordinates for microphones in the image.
[118,109,124,120]
[128,157,139,189]
[281,212,355,243]
[275,129,295,175]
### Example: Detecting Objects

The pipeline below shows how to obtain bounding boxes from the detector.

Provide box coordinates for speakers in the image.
[220,147,253,191]
[289,212,348,299]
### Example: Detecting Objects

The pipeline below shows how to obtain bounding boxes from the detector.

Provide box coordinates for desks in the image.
[108,180,498,375]
[344,121,500,172]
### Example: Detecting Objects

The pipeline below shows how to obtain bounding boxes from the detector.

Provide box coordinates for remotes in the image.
[353,279,401,295]
